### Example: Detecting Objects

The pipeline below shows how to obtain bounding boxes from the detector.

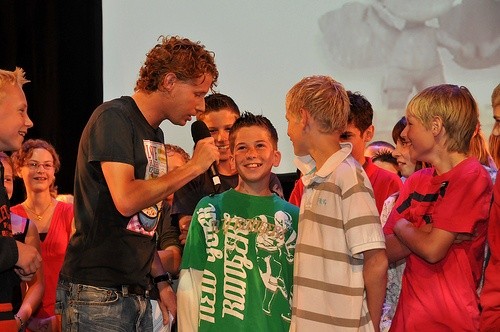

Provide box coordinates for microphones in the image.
[191,121,221,190]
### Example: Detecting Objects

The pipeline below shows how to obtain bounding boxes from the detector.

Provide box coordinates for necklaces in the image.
[25,197,52,221]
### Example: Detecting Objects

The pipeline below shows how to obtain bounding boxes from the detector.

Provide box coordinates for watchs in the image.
[151,271,170,285]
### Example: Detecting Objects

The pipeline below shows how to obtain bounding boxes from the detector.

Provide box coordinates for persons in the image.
[383,84,492,332]
[486,83,500,331]
[55,34,220,332]
[285,74,389,332]
[0,66,43,332]
[1,83,500,332]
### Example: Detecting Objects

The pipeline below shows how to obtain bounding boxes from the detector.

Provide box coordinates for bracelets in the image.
[15,314,24,327]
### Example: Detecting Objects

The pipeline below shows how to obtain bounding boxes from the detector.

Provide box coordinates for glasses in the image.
[23,159,54,170]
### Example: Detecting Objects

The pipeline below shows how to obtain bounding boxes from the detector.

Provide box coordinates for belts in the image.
[67,277,151,299]
